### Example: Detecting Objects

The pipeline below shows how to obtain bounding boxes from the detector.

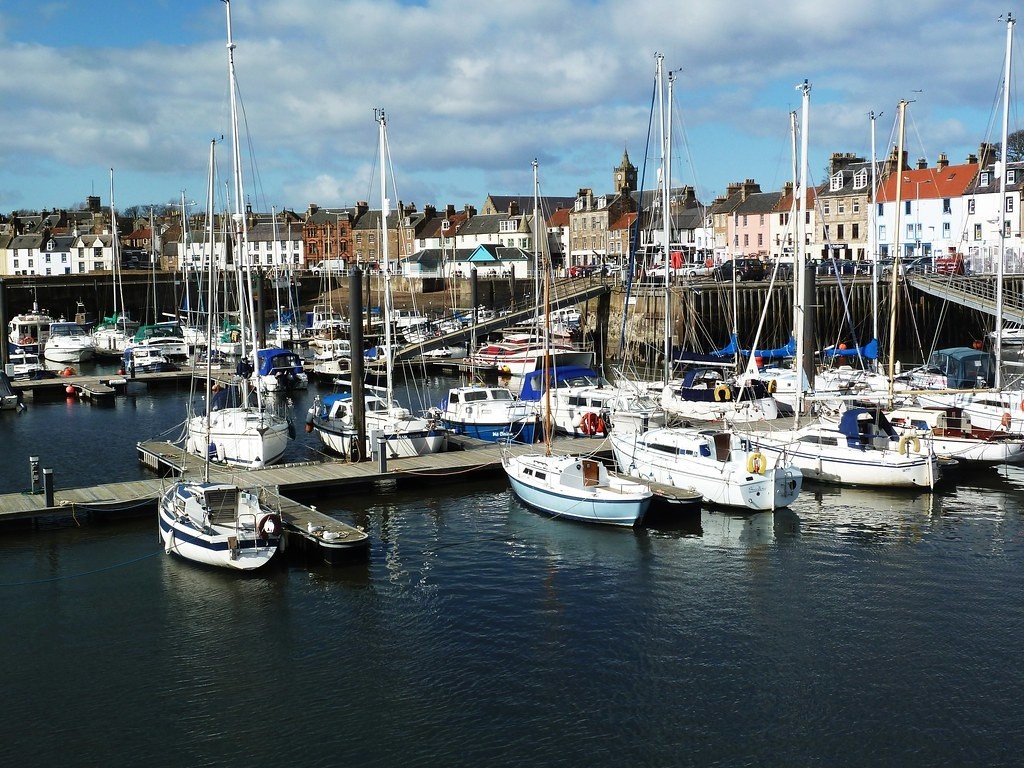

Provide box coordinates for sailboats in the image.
[157,135,292,573]
[496,157,655,527]
[7,13,1024,513]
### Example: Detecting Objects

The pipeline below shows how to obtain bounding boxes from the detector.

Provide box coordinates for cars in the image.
[684,263,716,277]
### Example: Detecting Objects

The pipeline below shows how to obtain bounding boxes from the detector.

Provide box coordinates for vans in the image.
[312,259,346,275]
[711,258,765,282]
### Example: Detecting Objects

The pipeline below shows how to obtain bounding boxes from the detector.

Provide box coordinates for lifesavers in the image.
[258,514,282,540]
[580,412,599,435]
[767,379,777,393]
[899,435,920,455]
[23,336,35,345]
[713,386,731,402]
[747,454,766,474]
